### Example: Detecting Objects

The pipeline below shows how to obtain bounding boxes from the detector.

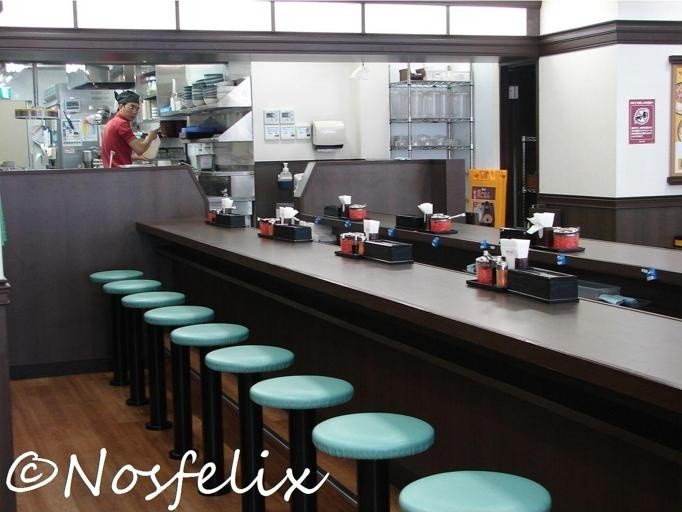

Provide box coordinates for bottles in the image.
[211,210,217,224]
[358,236,366,256]
[425,214,432,232]
[543,227,553,247]
[208,209,213,225]
[351,236,358,256]
[268,220,276,236]
[496,262,509,289]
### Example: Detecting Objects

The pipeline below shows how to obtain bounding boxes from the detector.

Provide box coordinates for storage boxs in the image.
[578,279,621,301]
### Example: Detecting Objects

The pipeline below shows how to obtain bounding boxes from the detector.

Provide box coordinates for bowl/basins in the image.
[430,213,452,232]
[260,216,274,236]
[340,233,365,254]
[201,85,217,105]
[551,227,580,251]
[203,73,224,85]
[474,257,505,285]
[183,85,195,109]
[216,80,236,101]
[191,79,206,107]
[349,204,368,220]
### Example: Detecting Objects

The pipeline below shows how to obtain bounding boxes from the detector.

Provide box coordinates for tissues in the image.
[216,199,245,230]
[324,194,352,216]
[527,211,561,248]
[273,205,313,243]
[362,219,413,265]
[499,238,580,305]
[395,202,434,227]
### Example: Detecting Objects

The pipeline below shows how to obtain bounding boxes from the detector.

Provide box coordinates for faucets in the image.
[107,150,117,168]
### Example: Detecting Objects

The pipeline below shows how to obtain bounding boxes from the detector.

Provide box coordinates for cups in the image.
[158,160,171,167]
[390,88,471,120]
[393,135,460,147]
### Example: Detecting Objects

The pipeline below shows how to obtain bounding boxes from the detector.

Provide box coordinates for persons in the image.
[102,91,157,168]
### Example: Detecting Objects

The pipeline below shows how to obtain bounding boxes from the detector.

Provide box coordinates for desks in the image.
[134,221,681,512]
[303,210,680,308]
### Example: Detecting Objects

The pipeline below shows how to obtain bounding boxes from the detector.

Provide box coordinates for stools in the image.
[169,323,250,496]
[89,270,144,386]
[312,412,437,511]
[399,471,552,512]
[144,305,213,459]
[120,291,185,431]
[249,374,354,512]
[205,345,295,512]
[102,279,162,405]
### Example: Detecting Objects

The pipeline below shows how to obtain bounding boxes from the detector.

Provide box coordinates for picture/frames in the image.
[64,99,81,113]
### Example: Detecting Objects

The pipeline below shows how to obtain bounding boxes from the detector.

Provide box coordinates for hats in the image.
[117,91,139,104]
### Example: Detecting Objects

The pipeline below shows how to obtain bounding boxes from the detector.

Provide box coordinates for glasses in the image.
[120,104,139,112]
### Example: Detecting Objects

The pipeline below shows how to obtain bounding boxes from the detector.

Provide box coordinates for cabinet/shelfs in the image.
[133,63,185,122]
[389,60,475,169]
[159,78,251,142]
